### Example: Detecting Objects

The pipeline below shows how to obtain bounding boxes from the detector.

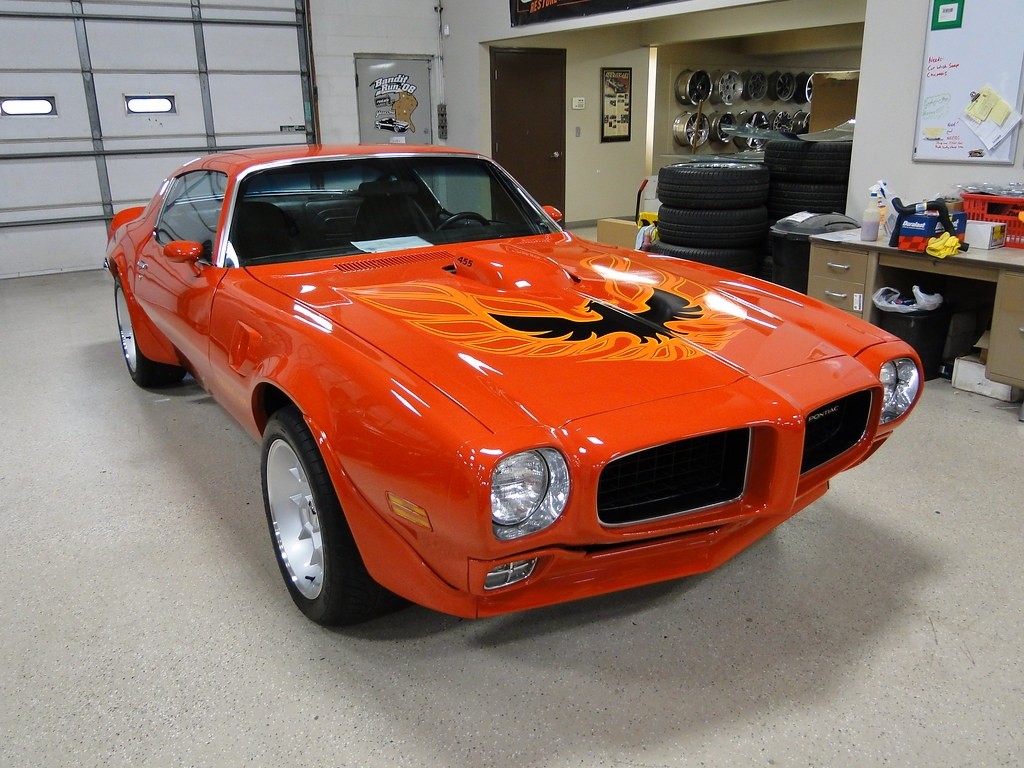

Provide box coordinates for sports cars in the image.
[103,145,926,630]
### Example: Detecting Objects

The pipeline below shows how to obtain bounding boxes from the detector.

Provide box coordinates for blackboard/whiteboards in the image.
[911,1,1023,166]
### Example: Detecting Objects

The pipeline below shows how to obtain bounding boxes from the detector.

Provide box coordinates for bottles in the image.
[860,194,881,242]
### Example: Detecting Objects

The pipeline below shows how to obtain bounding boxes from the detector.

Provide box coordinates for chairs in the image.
[229,201,304,259]
[354,194,434,243]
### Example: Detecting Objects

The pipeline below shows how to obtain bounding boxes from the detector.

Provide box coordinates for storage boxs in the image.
[952,354,1024,403]
[796,71,859,141]
[972,330,991,363]
[644,199,662,214]
[596,218,638,249]
[965,220,1007,249]
[943,200,964,211]
[960,193,1024,248]
[644,176,658,200]
[897,211,967,252]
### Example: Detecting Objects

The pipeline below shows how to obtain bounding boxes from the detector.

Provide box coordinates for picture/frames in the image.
[600,66,633,143]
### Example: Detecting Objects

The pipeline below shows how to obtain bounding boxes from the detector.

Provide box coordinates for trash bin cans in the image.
[878,311,951,381]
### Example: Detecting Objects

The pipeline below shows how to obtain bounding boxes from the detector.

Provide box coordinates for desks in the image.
[807,224,1024,392]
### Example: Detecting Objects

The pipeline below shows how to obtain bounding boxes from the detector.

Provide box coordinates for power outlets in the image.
[572,97,585,109]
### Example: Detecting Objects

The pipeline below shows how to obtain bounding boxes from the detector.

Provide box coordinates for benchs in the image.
[178,198,358,250]
